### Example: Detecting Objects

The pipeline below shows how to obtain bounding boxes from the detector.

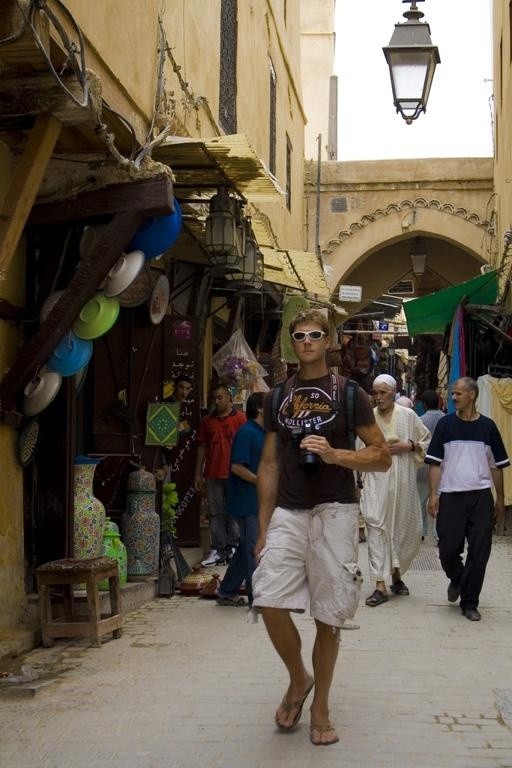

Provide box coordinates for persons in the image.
[166,379,192,472]
[425,375,511,621]
[215,392,266,606]
[396,390,414,409]
[355,374,431,606]
[250,308,394,746]
[193,383,247,571]
[416,390,447,546]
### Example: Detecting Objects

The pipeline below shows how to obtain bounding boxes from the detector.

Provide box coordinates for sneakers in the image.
[201,548,225,568]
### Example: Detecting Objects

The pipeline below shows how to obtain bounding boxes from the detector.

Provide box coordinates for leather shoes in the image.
[447,582,460,602]
[459,602,482,621]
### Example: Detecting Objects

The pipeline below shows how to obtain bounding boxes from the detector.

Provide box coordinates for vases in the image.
[72,454,108,593]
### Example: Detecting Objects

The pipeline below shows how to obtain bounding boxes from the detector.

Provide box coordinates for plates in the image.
[124,197,182,258]
[103,249,145,297]
[150,275,170,324]
[21,372,63,417]
[70,291,120,340]
[118,267,155,308]
[49,330,93,377]
[16,418,43,468]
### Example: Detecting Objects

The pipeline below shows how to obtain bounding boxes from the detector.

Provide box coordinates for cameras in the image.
[290,420,325,476]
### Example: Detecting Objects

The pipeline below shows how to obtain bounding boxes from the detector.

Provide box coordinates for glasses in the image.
[291,331,327,342]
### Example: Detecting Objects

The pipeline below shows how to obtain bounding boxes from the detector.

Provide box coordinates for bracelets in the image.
[409,440,415,454]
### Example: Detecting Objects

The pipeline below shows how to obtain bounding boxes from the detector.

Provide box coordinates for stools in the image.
[31,555,126,651]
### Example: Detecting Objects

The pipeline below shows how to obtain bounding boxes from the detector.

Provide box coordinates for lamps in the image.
[410,237,428,285]
[381,3,443,129]
[238,251,265,297]
[204,184,236,254]
[208,222,243,276]
[225,231,257,287]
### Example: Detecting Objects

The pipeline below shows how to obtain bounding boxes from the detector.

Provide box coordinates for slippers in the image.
[309,722,339,746]
[365,589,390,606]
[390,581,410,596]
[274,678,314,730]
[216,597,247,606]
